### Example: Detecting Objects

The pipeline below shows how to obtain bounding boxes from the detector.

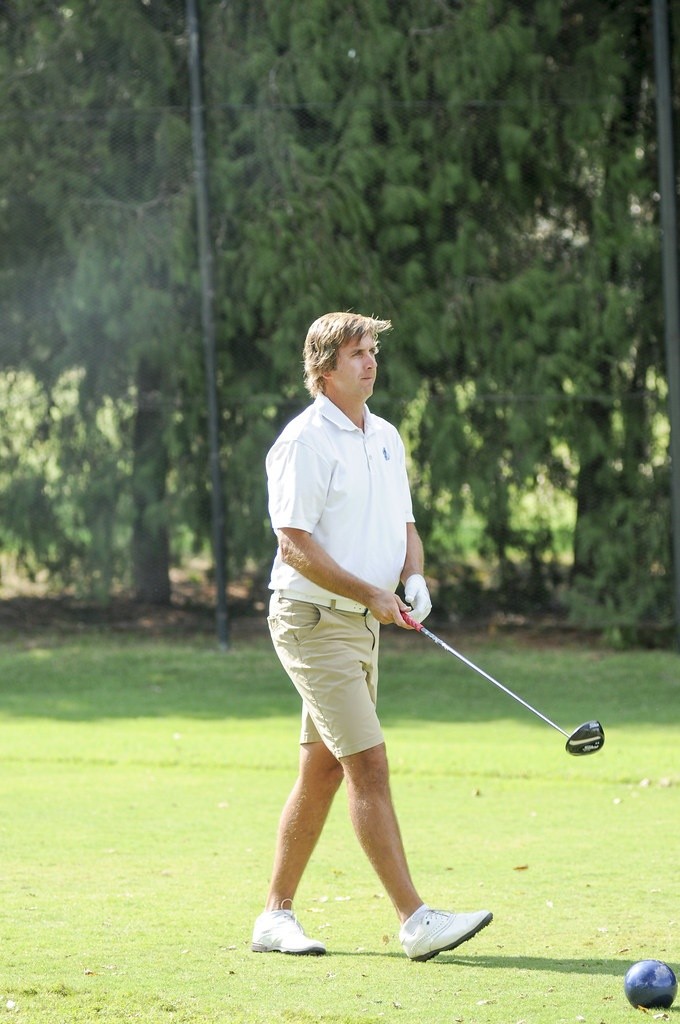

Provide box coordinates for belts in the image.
[273,589,370,618]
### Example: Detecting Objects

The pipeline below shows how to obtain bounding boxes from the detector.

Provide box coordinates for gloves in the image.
[398,574,432,629]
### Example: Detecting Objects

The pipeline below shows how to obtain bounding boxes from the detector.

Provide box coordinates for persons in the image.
[249,312,495,960]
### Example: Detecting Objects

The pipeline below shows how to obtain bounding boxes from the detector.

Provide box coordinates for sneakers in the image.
[251,899,326,955]
[402,909,493,961]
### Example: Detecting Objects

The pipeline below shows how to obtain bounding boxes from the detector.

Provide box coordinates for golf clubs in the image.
[400,610,606,756]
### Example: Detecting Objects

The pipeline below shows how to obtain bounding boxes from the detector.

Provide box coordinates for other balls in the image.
[624,959,677,1010]
